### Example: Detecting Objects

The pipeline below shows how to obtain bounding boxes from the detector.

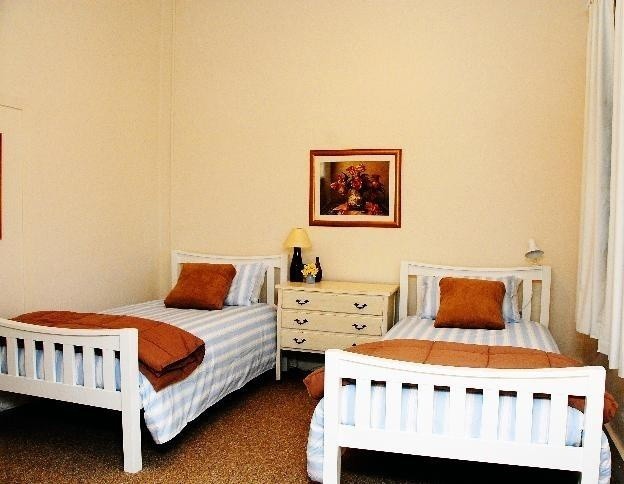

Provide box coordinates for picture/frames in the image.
[309,149,401,228]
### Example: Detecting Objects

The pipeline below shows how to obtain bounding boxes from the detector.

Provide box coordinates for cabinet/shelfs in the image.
[275,280,400,381]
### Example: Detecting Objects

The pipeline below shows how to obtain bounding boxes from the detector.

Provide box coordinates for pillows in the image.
[164,262,269,309]
[419,275,522,330]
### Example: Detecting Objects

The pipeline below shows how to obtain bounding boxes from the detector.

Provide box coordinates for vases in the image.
[306,274,315,284]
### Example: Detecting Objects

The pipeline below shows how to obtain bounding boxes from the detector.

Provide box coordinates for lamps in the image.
[283,228,312,281]
[525,237,543,266]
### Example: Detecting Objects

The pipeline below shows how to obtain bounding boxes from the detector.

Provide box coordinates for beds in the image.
[323,261,606,483]
[0,249,290,475]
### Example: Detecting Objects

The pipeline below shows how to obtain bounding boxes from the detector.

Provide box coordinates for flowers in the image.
[301,262,319,279]
